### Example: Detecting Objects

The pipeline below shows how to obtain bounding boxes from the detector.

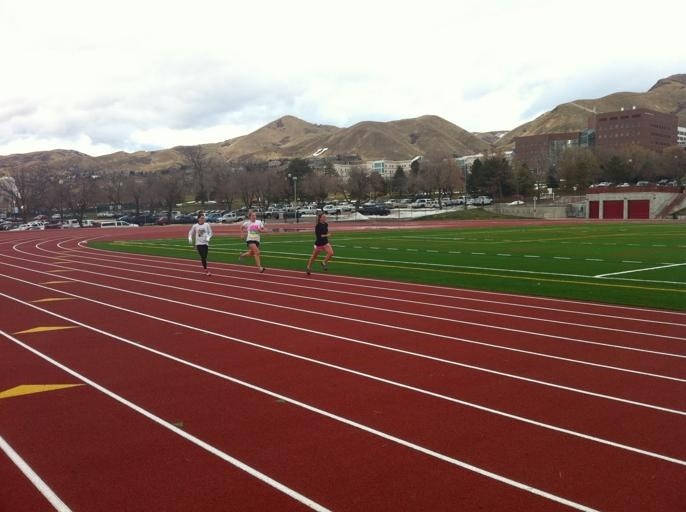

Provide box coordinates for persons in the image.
[305,211,334,275]
[238,210,265,273]
[188,210,214,276]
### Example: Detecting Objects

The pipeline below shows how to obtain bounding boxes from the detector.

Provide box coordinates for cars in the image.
[588,179,686,188]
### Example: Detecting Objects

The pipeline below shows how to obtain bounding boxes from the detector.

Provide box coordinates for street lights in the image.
[288,174,297,222]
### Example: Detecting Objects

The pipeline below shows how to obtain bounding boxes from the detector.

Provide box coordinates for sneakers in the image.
[239,252,242,260]
[306,264,311,274]
[257,267,265,273]
[321,261,327,271]
[202,269,210,275]
